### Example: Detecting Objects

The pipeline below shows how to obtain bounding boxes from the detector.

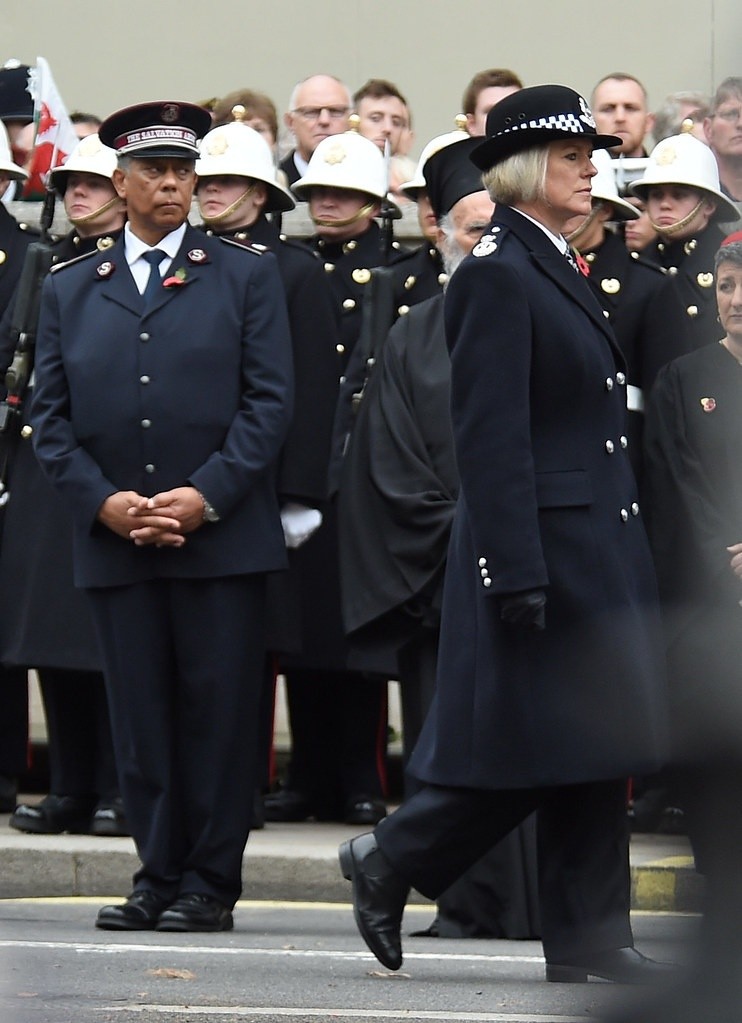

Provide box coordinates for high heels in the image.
[264,786,314,822]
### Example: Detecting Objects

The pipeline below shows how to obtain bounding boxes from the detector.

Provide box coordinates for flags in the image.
[22,55,80,198]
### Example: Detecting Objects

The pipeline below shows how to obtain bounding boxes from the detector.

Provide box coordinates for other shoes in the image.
[408,917,476,937]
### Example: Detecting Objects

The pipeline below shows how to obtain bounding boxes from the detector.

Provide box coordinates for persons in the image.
[339,134,547,942]
[640,239,741,988]
[261,112,400,826]
[192,105,348,668]
[28,101,297,931]
[0,57,742,989]
[628,118,741,342]
[590,73,650,159]
[278,74,356,188]
[338,83,681,989]
[46,131,129,275]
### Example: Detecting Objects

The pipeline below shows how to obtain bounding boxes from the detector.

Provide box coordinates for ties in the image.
[141,249,168,304]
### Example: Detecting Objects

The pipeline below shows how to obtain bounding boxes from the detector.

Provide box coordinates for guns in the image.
[0,120,629,495]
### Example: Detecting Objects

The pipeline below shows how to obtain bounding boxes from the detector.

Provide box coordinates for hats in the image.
[470,84,623,177]
[0,66,34,120]
[423,133,487,225]
[98,101,212,158]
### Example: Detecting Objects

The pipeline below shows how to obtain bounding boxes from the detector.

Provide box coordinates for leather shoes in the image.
[96,891,164,931]
[338,832,411,971]
[157,894,233,932]
[315,790,387,824]
[9,794,89,834]
[546,946,690,985]
[91,798,131,836]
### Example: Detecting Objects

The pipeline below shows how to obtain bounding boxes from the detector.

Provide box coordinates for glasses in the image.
[671,108,714,136]
[291,104,349,118]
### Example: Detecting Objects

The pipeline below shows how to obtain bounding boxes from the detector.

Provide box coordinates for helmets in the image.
[590,149,641,219]
[398,130,471,202]
[0,122,29,180]
[628,132,741,223]
[193,105,295,213]
[290,114,403,220]
[45,133,118,192]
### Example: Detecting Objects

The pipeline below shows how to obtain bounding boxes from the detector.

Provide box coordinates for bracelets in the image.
[198,491,219,523]
